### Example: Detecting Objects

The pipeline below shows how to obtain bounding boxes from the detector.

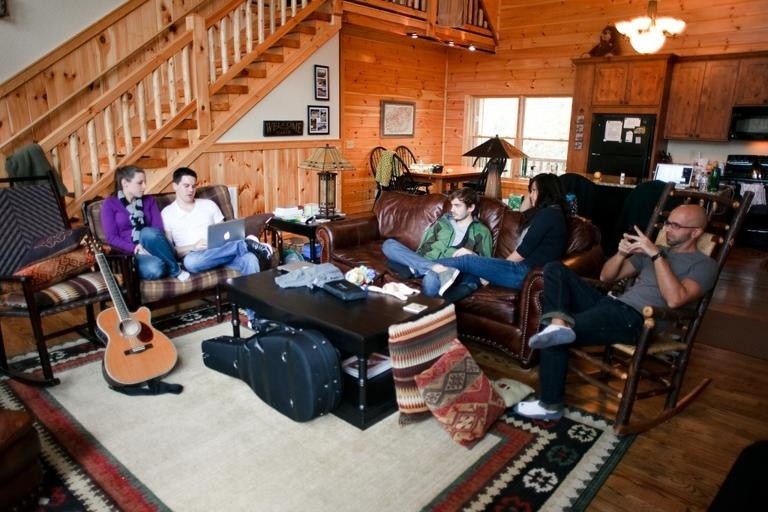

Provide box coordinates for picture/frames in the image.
[308,105,330,135]
[379,99,415,138]
[315,64,329,100]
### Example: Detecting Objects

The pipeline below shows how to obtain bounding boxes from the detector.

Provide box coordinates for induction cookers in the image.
[719,174,768,182]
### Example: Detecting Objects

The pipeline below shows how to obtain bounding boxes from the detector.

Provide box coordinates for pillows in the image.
[491,378,535,407]
[413,338,506,450]
[13,244,111,292]
[388,304,459,427]
[19,225,90,269]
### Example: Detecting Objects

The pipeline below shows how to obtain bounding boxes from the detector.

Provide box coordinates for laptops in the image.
[191,217,245,252]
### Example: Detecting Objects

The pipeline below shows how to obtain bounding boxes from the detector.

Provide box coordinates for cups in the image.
[304,204,311,216]
[509,196,522,212]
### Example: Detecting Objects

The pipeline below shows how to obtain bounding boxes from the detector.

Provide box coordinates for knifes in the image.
[658,150,668,159]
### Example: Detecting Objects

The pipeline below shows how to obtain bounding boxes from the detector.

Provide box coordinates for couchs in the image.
[81,184,283,323]
[315,193,605,369]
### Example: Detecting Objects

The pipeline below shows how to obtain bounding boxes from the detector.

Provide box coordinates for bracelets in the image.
[652,251,667,262]
[638,242,640,245]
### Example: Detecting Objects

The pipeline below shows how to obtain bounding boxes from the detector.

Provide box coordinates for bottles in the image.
[719,161,725,176]
[593,171,601,185]
[709,169,718,192]
[619,172,625,184]
[700,176,709,192]
[695,167,704,189]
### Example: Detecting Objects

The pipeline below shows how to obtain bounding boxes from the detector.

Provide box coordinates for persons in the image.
[160,167,261,330]
[385,173,571,303]
[513,204,719,421]
[101,165,190,282]
[381,188,494,296]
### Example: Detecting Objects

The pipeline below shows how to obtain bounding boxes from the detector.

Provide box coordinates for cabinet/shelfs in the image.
[567,49,768,182]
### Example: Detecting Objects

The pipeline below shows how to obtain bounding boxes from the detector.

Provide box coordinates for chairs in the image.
[395,146,433,196]
[0,172,133,387]
[370,147,387,210]
[568,181,754,436]
[382,154,425,195]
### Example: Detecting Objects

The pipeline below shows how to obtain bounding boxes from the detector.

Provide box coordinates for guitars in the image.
[84,234,178,390]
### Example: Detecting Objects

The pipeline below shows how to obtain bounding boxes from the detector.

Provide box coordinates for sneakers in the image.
[437,266,461,297]
[245,234,274,261]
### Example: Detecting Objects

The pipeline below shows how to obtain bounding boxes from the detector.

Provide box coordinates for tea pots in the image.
[433,165,443,173]
[750,158,766,180]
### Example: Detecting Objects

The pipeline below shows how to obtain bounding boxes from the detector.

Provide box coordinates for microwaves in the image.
[586,113,657,179]
[728,107,768,141]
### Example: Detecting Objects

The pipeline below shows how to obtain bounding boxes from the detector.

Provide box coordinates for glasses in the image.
[662,219,703,233]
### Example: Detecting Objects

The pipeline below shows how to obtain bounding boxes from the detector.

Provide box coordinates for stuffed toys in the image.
[581,26,621,58]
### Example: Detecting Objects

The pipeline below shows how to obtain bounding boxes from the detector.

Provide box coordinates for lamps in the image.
[614,0,686,56]
[298,143,356,220]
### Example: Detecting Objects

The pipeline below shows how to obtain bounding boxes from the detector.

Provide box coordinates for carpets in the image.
[1,303,638,510]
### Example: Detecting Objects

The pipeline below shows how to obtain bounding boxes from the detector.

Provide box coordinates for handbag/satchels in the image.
[325,278,367,301]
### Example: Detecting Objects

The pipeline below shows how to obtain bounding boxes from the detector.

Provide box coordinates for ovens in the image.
[718,182,768,234]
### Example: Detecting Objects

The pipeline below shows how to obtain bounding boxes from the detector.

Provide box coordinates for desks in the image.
[402,163,486,195]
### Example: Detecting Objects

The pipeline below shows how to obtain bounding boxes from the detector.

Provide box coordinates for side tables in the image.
[268,216,318,263]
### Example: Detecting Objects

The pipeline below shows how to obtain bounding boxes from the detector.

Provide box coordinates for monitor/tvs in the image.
[654,163,693,190]
